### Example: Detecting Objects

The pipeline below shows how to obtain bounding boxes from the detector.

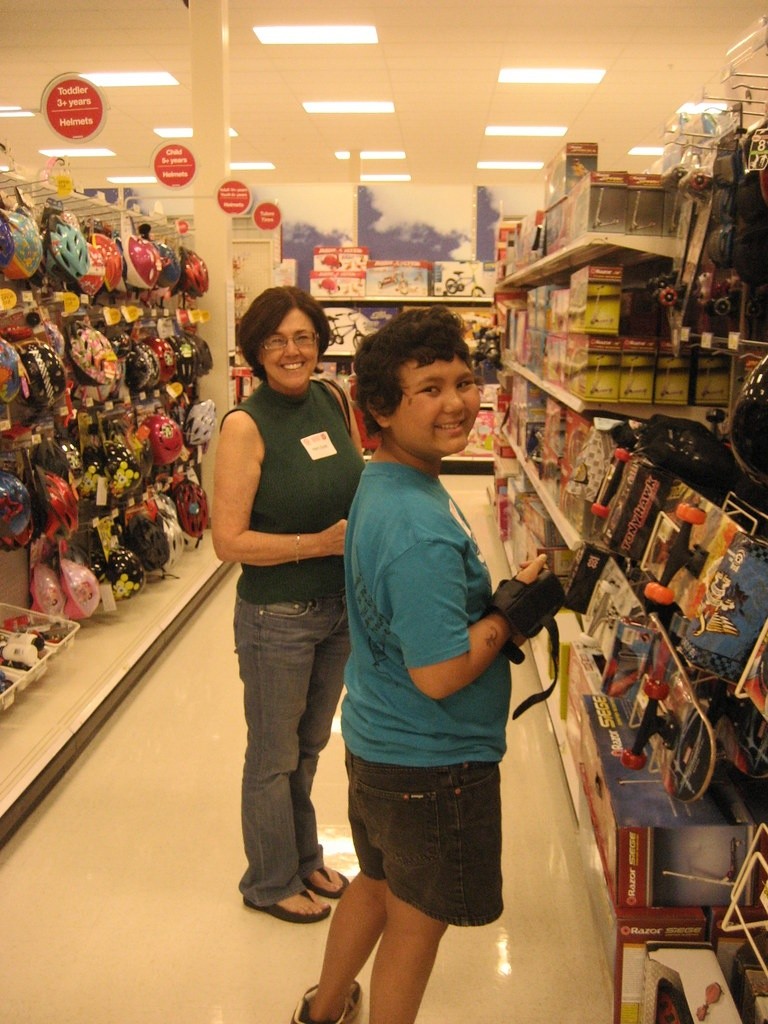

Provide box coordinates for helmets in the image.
[0,208,215,618]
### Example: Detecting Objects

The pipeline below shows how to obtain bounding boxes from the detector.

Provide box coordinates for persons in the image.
[291,306,550,1024]
[210,286,369,922]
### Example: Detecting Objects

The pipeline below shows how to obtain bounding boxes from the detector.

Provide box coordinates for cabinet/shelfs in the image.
[311,293,496,464]
[483,229,768,1023]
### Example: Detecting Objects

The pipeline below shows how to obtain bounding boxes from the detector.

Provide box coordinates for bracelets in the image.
[294,535,303,563]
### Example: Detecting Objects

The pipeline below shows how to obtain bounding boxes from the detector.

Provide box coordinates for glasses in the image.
[260,330,319,351]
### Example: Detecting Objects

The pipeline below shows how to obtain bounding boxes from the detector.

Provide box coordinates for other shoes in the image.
[289,977,362,1023]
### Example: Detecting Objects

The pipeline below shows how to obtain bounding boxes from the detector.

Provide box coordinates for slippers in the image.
[304,865,348,898]
[242,887,333,923]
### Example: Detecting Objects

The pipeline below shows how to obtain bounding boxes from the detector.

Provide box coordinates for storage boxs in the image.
[488,141,767,1024]
[309,245,493,459]
